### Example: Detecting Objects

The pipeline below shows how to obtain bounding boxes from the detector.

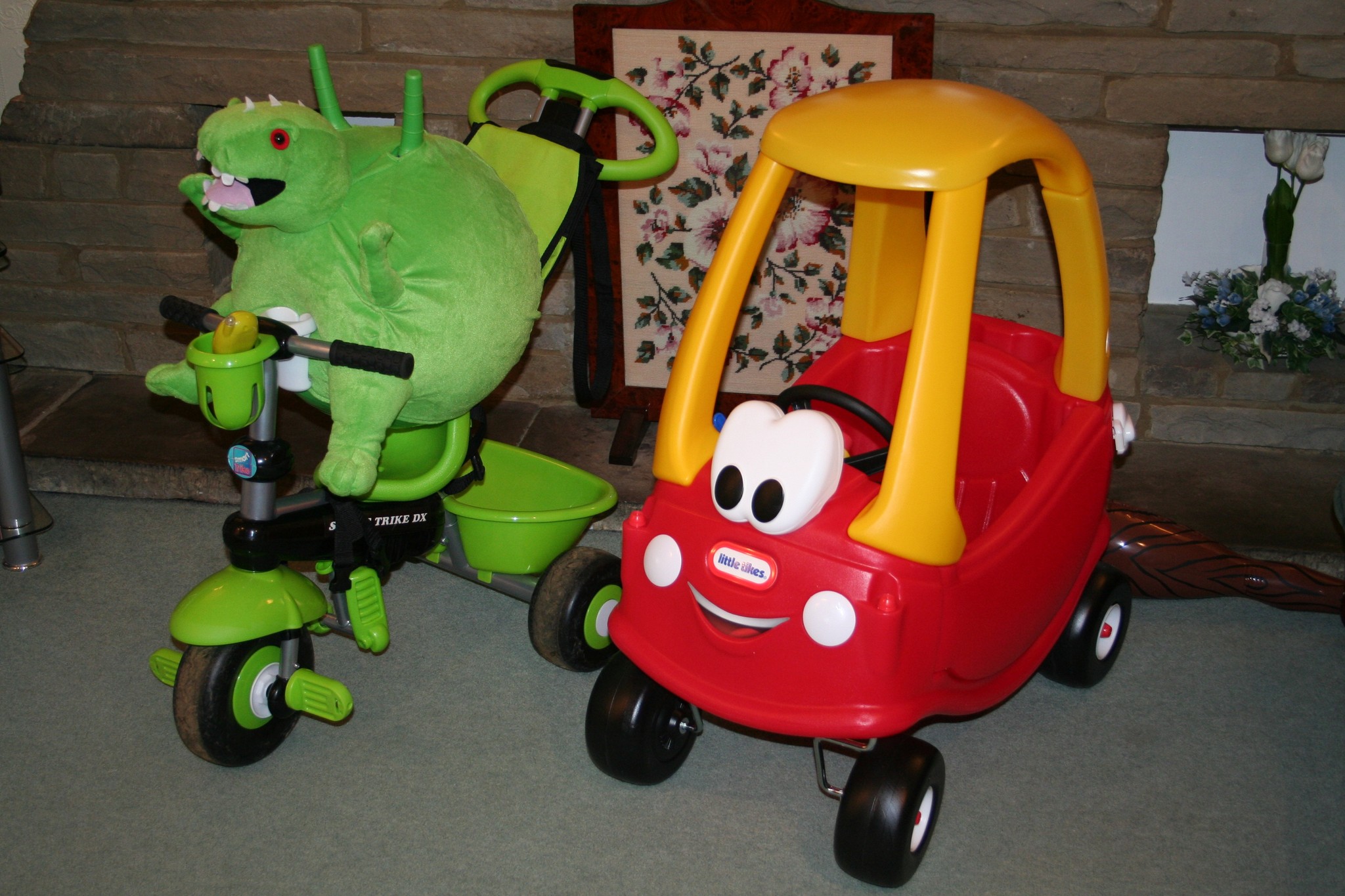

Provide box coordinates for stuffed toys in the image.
[142,94,545,502]
